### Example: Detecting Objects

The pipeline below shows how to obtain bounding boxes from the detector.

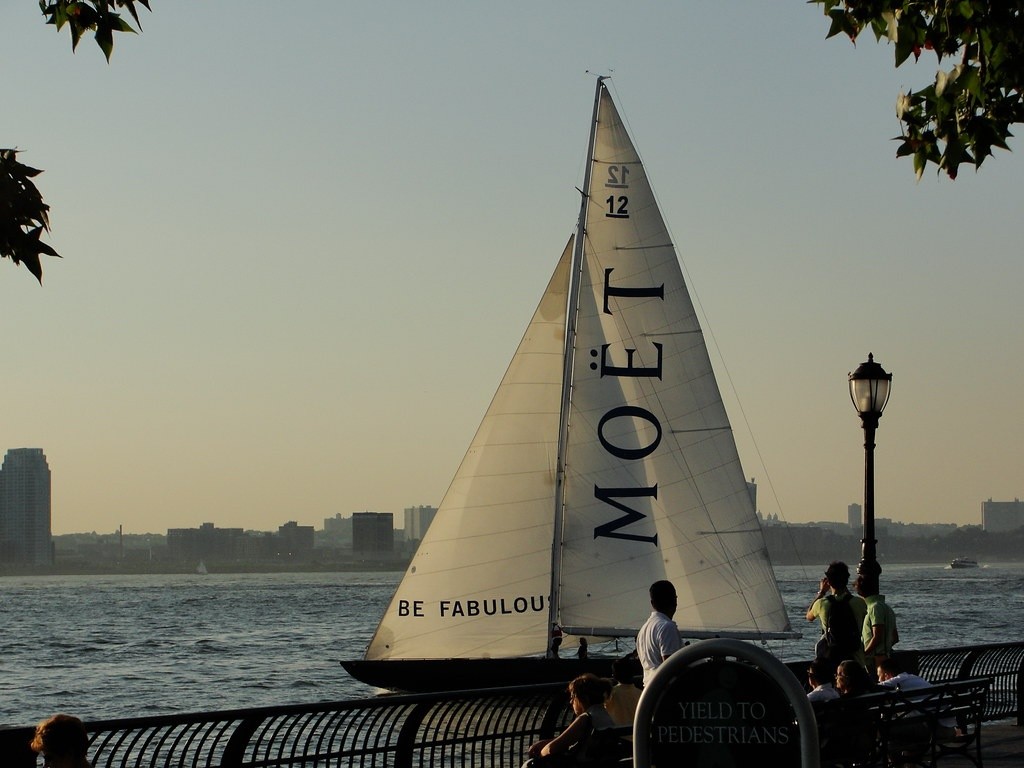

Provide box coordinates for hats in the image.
[824,561,851,580]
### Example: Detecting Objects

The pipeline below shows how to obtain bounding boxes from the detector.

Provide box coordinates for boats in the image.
[950,556,980,570]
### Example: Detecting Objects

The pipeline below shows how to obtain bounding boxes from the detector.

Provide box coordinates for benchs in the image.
[826,677,996,768]
[549,698,826,768]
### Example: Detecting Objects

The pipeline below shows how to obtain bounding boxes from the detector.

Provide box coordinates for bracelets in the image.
[818,591,826,596]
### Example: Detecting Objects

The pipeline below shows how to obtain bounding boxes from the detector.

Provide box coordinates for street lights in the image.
[848,351,895,576]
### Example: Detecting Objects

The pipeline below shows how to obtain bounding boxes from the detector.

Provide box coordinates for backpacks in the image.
[825,593,859,642]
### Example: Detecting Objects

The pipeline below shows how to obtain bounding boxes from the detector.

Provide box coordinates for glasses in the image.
[807,670,812,677]
[834,673,846,678]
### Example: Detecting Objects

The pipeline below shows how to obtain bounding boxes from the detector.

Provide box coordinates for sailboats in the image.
[338,66,922,702]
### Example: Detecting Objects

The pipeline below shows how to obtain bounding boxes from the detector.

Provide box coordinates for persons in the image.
[30,714,93,768]
[521,581,692,768]
[805,559,958,768]
